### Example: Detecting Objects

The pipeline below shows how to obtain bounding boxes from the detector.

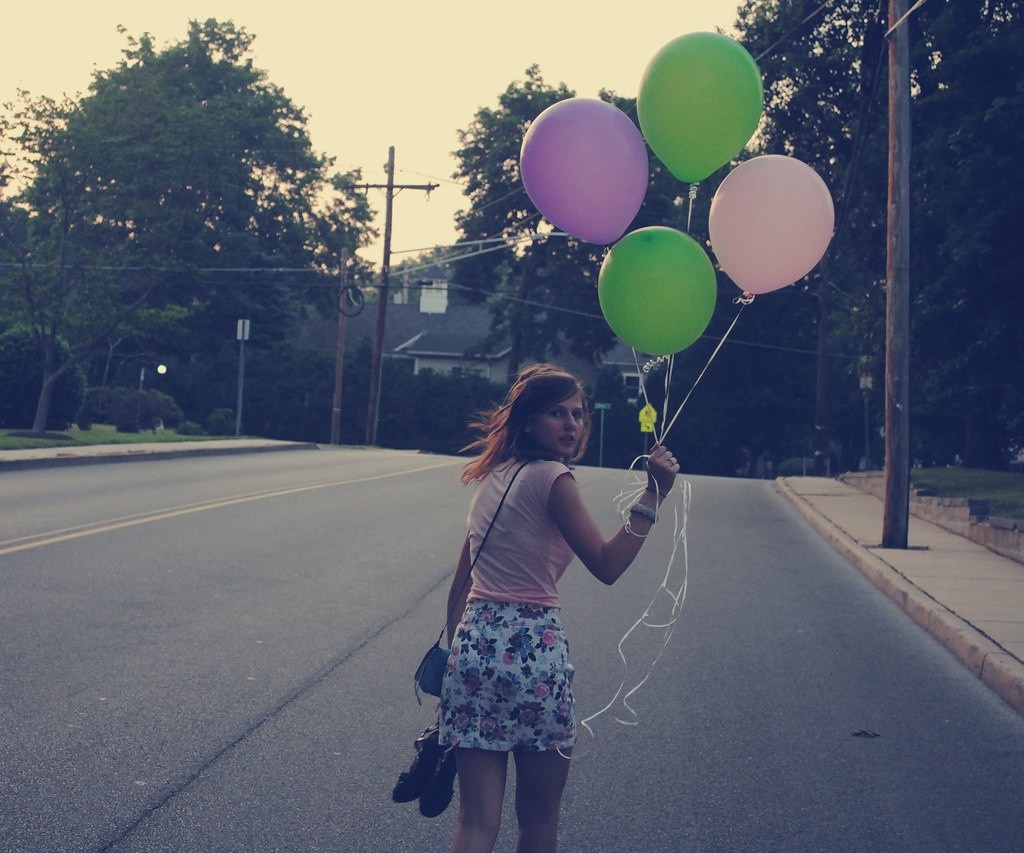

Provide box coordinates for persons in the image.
[438,362,681,853]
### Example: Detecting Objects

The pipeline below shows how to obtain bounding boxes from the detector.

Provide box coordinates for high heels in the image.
[393,723,458,817]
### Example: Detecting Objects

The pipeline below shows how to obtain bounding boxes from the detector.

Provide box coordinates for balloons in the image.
[520,97,649,244]
[708,154,836,293]
[638,32,764,186]
[599,227,719,359]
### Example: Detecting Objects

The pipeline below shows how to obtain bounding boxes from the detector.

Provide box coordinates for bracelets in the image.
[629,504,659,523]
[645,487,666,500]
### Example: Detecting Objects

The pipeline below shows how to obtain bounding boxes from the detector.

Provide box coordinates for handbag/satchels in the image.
[414,640,451,697]
